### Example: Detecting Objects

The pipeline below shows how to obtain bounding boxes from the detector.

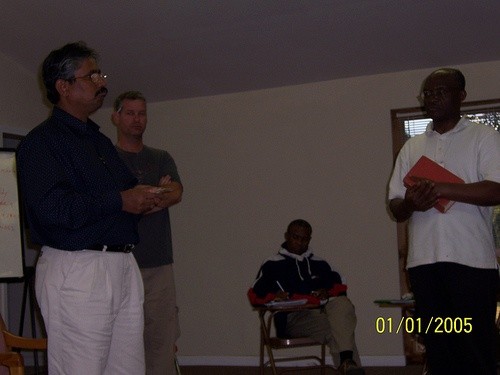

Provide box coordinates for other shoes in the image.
[336,357,365,375]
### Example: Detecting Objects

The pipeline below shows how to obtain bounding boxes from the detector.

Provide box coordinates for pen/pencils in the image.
[276,280,290,301]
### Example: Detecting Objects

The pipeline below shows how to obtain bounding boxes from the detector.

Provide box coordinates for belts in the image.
[83,242,136,253]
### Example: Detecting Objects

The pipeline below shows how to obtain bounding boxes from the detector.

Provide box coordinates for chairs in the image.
[0,314,48,375]
[253,303,326,375]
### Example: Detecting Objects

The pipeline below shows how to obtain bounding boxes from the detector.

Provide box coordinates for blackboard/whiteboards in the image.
[0,148,26,282]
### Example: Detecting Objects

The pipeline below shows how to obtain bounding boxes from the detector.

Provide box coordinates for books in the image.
[403,155,464,213]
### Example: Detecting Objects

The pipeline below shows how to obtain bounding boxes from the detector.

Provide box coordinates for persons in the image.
[388,69,500,375]
[15,41,164,375]
[248,220,360,375]
[111,91,185,375]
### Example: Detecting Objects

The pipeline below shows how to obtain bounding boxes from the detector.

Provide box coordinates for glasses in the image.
[70,71,109,84]
